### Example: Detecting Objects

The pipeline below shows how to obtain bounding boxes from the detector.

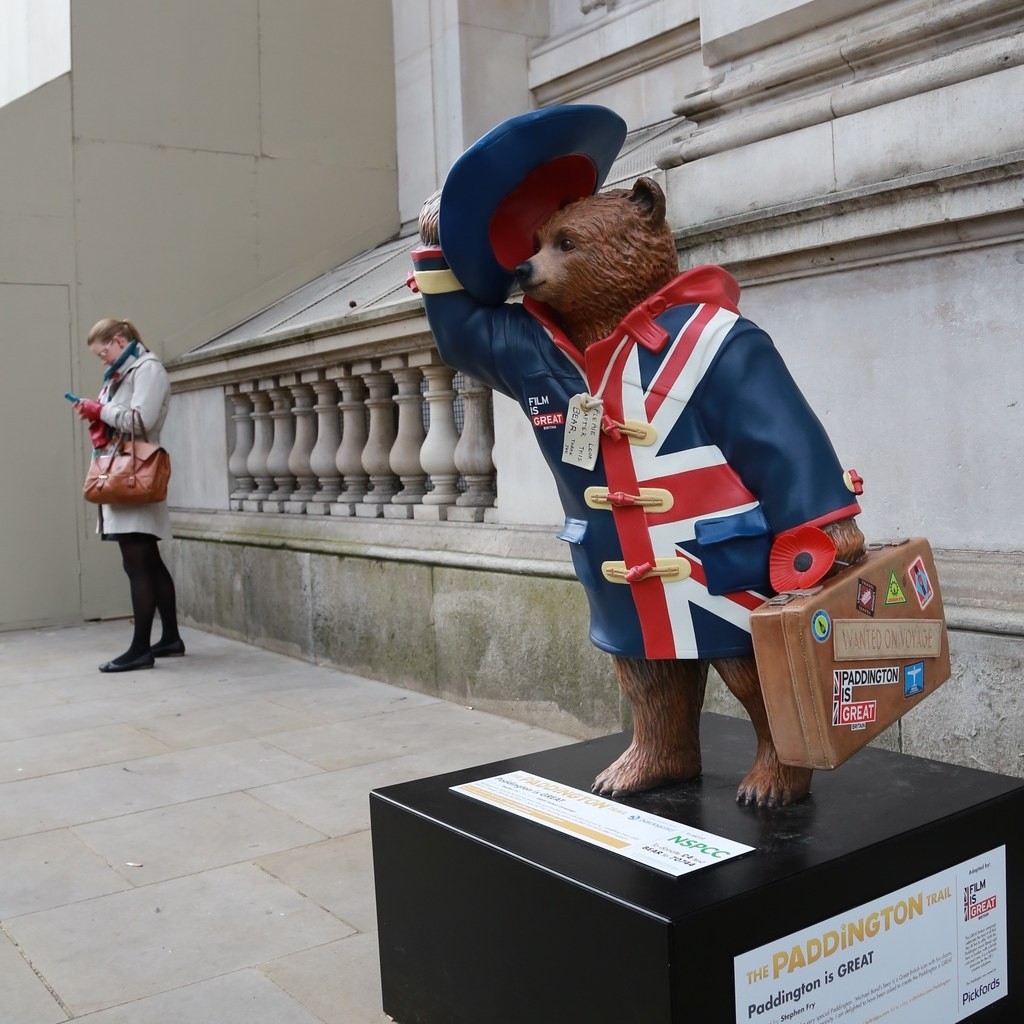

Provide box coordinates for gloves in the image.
[83,401,102,422]
[89,420,109,448]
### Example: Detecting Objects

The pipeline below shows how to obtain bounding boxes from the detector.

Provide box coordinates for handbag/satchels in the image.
[82,410,171,503]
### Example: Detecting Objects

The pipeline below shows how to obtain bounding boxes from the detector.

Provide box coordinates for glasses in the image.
[98,339,112,358]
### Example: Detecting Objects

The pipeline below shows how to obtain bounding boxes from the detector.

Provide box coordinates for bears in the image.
[412,176,868,814]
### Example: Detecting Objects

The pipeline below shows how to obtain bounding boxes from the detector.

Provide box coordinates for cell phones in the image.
[65,392,80,402]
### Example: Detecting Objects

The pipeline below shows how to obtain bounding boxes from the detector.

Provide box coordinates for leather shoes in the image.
[98,652,154,672]
[151,640,185,655]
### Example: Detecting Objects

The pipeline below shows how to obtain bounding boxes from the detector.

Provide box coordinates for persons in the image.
[72,319,185,673]
